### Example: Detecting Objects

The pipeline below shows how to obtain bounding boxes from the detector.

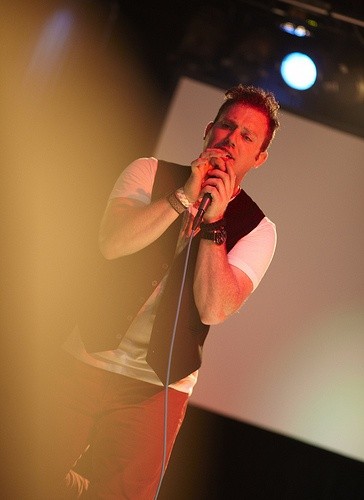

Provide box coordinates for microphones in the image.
[192,192,212,231]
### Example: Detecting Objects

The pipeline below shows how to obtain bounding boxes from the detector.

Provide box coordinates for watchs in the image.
[199,229,228,246]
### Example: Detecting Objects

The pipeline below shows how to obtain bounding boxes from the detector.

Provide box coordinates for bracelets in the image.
[175,186,193,208]
[200,218,226,231]
[166,192,186,215]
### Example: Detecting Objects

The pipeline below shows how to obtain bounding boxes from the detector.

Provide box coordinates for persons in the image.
[11,82,279,500]
